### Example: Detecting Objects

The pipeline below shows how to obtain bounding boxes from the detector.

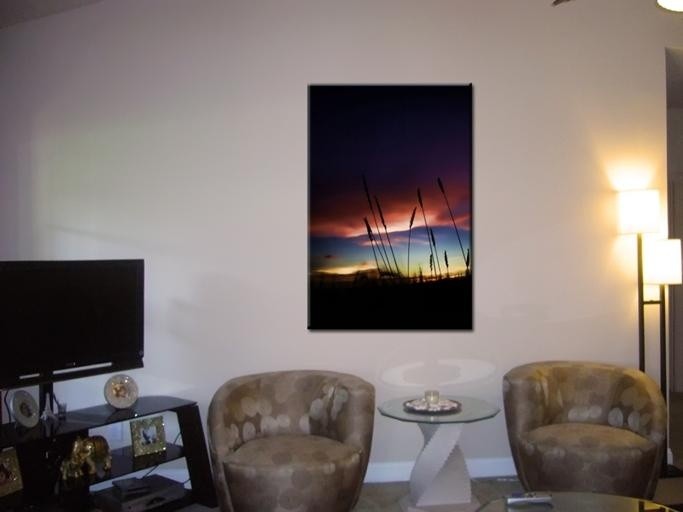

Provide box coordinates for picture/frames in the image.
[128,415,169,459]
[0,445,24,496]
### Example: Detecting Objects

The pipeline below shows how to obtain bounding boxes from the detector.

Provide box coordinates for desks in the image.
[377,394,500,512]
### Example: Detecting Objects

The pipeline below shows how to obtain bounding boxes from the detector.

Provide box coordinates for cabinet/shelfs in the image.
[0,394,215,512]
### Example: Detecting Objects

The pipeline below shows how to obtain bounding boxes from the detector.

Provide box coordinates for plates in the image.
[403,398,461,413]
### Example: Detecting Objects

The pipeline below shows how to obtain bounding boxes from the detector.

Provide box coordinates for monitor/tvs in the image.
[0,259,144,391]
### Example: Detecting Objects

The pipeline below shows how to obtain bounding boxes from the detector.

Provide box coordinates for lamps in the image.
[612,185,682,478]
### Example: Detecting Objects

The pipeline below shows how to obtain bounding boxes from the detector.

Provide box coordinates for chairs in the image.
[501,361,669,500]
[209,370,377,512]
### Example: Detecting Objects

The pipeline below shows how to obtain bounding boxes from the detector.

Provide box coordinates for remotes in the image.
[504,493,553,507]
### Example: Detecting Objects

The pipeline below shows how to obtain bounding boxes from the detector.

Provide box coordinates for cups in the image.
[423,392,440,410]
